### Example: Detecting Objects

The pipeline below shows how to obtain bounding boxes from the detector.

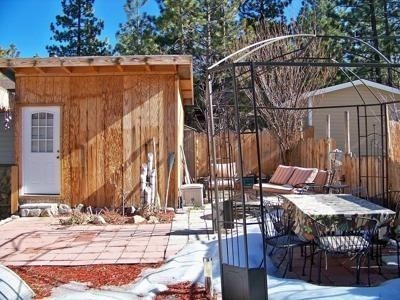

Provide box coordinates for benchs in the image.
[252,163,319,199]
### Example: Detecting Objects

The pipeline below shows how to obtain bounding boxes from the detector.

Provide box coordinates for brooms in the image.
[164,151,175,212]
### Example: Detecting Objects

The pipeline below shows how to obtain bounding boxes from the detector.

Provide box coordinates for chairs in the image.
[203,159,236,196]
[254,182,400,288]
